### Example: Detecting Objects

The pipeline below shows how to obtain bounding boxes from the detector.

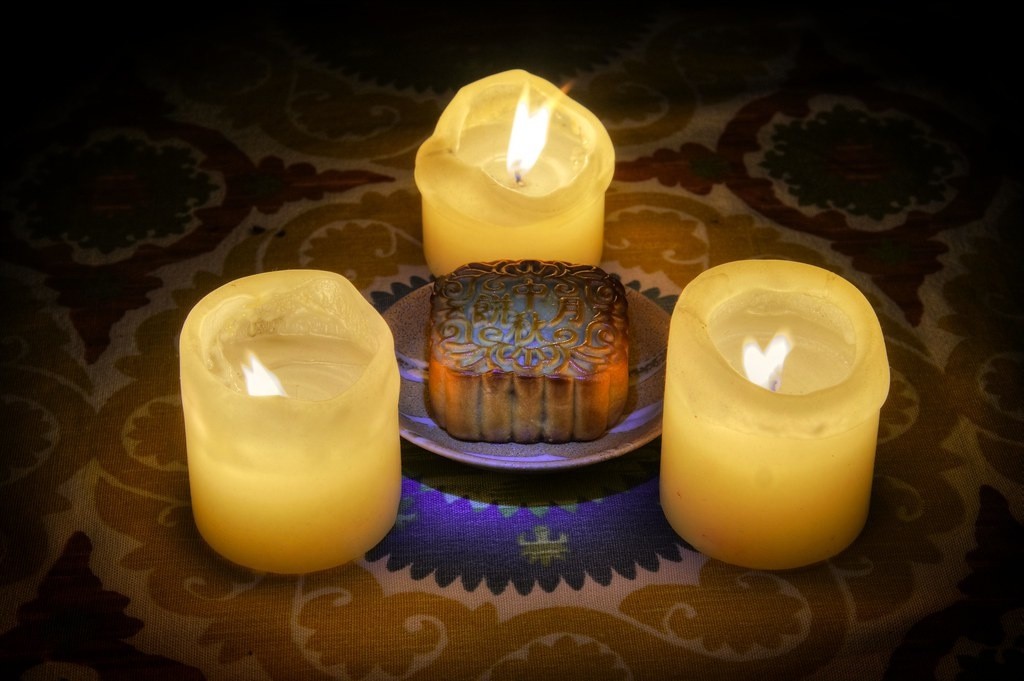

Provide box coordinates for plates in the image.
[382,277,670,471]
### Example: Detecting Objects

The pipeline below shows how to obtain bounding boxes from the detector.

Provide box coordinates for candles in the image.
[414,68,614,279]
[661,258,891,568]
[178,268,401,570]
